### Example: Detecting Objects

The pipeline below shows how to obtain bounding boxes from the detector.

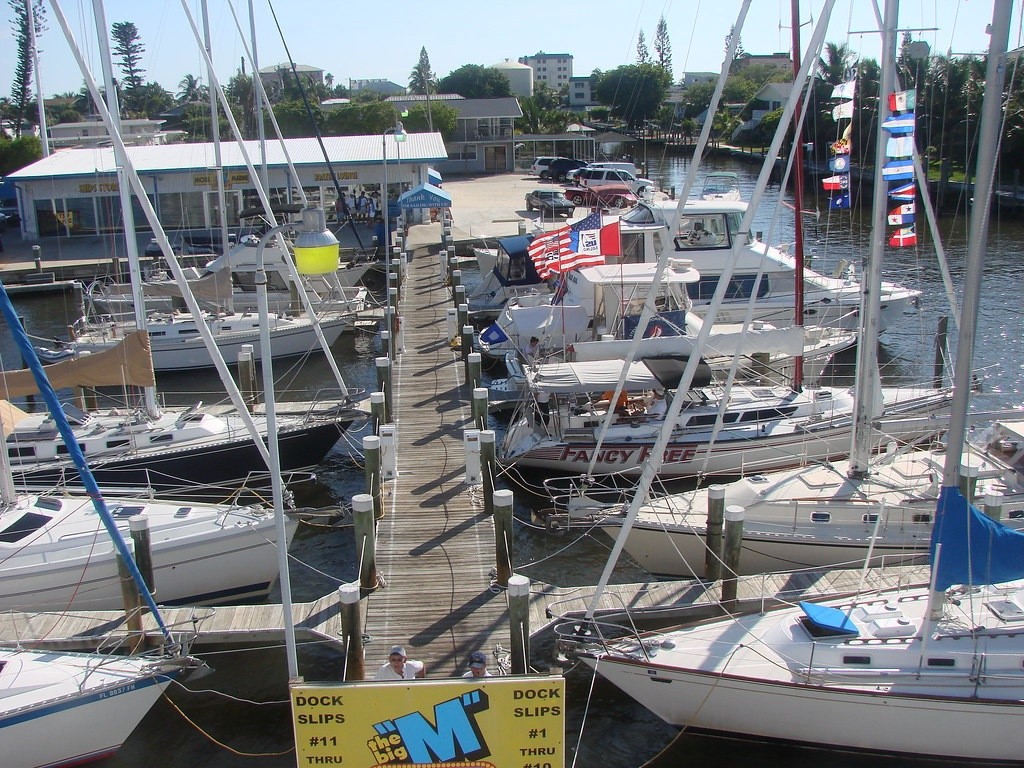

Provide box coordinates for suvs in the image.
[527,156,654,208]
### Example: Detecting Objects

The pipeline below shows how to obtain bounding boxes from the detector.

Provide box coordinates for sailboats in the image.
[0,0,379,614]
[463,0,1024,768]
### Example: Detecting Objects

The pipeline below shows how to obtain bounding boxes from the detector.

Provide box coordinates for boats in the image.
[699,171,741,203]
[0,284,216,768]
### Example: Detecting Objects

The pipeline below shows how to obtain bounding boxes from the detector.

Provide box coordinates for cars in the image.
[525,189,575,218]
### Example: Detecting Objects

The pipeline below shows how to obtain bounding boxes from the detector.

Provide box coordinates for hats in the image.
[390,645,407,657]
[652,389,664,395]
[469,651,487,668]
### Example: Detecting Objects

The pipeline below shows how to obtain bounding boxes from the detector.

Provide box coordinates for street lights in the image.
[382,121,408,369]
[395,104,408,231]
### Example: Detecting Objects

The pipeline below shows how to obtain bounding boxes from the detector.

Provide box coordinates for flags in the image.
[822,60,860,210]
[881,88,919,247]
[527,211,606,279]
[568,221,623,257]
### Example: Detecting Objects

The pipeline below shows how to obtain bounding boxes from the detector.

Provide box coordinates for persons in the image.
[370,644,426,681]
[592,389,628,410]
[642,389,666,423]
[336,191,379,230]
[524,337,542,362]
[535,391,552,428]
[463,651,492,678]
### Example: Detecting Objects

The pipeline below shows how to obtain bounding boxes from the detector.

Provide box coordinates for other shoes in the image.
[547,434,552,437]
